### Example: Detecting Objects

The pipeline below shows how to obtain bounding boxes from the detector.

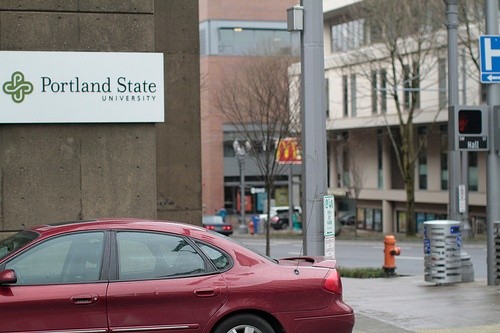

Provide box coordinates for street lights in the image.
[233,139,252,233]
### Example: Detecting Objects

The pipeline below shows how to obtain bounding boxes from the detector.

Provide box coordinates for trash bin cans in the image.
[422,219,466,285]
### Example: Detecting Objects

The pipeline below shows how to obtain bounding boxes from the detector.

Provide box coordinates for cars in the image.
[0,217,356,333]
[202,215,234,237]
[270,212,288,231]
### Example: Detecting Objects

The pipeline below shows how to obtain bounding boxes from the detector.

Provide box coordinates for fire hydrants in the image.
[380,234,401,275]
